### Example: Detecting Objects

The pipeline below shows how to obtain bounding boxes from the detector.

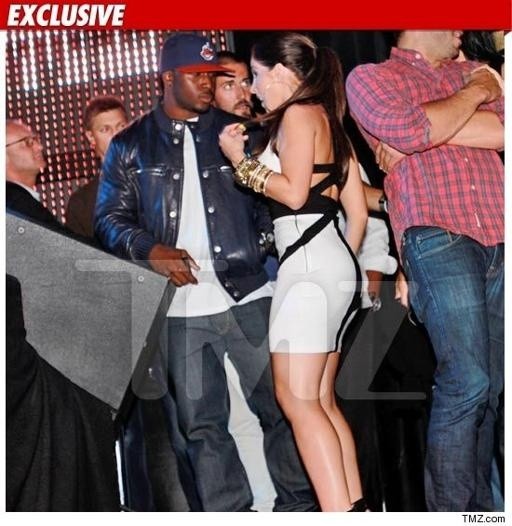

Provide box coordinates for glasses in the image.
[7,134,41,147]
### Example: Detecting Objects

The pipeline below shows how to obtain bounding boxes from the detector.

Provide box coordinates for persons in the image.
[6,31,505,512]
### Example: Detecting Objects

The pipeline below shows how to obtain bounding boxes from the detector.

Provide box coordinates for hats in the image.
[160,34,234,73]
